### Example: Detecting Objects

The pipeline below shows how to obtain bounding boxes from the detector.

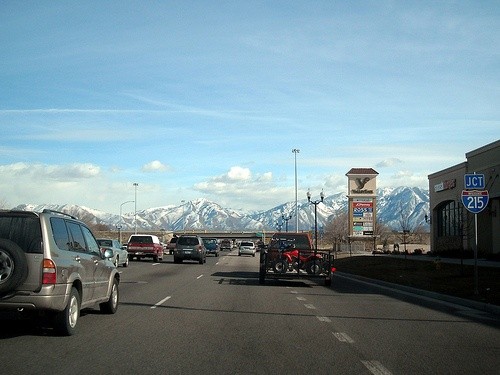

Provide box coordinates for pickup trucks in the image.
[260,232,335,285]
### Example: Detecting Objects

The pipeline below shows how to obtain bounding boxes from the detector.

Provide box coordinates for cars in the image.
[239,241,256,257]
[167,236,178,255]
[219,239,239,251]
[255,242,261,252]
[98,239,129,268]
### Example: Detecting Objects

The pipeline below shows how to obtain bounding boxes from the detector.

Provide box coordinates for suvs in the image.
[127,235,163,262]
[202,238,220,257]
[174,235,207,264]
[0,209,121,337]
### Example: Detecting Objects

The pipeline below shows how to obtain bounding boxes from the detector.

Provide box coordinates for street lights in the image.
[292,148,300,233]
[306,186,325,250]
[282,213,293,232]
[120,201,135,226]
[133,183,139,234]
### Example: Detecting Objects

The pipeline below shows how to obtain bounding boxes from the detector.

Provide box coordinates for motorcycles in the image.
[272,237,323,276]
